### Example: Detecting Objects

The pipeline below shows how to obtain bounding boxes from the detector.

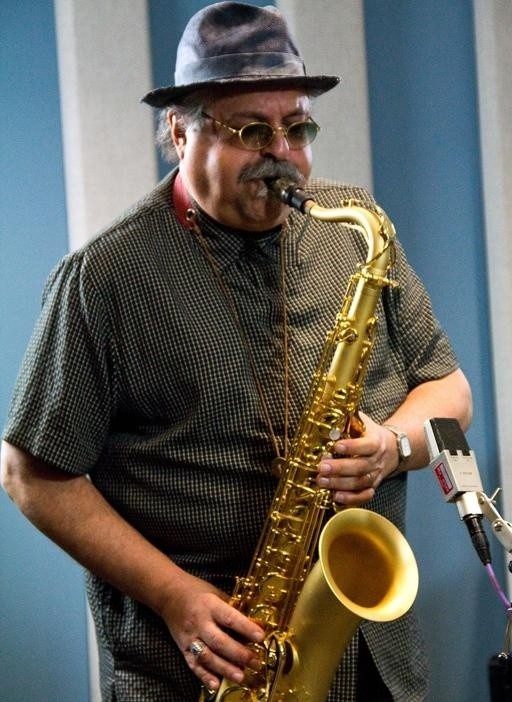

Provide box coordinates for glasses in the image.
[203,109,319,152]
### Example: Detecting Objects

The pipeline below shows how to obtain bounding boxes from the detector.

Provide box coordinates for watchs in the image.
[380,422,412,481]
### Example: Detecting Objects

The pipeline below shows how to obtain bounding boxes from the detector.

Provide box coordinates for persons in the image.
[1,2,477,702]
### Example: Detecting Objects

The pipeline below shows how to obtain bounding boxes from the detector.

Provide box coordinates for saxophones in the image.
[199,177,420,701]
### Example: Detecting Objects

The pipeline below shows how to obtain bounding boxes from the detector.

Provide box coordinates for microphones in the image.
[426,419,493,565]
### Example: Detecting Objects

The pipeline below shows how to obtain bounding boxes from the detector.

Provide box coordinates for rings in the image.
[189,640,206,661]
[366,473,374,485]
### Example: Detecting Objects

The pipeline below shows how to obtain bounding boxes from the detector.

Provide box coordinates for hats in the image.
[140,2,341,109]
